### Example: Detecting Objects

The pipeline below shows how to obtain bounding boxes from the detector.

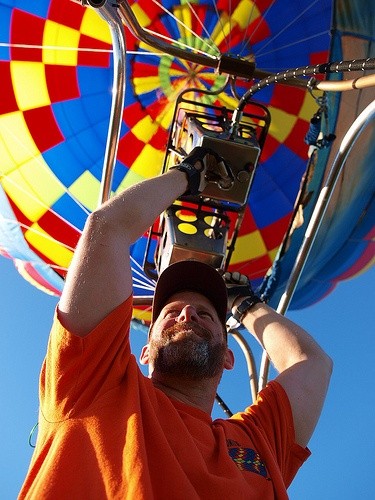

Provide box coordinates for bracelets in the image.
[233,295,263,323]
[230,292,252,312]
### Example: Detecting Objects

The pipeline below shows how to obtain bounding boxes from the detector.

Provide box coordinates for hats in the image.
[148,260,228,339]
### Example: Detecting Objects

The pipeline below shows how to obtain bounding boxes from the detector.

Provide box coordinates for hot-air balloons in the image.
[0,0,375,329]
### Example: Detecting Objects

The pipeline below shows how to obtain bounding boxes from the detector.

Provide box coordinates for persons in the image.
[12,146,333,499]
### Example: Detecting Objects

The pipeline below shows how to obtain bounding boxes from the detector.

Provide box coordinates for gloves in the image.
[215,267,251,311]
[171,145,235,196]
